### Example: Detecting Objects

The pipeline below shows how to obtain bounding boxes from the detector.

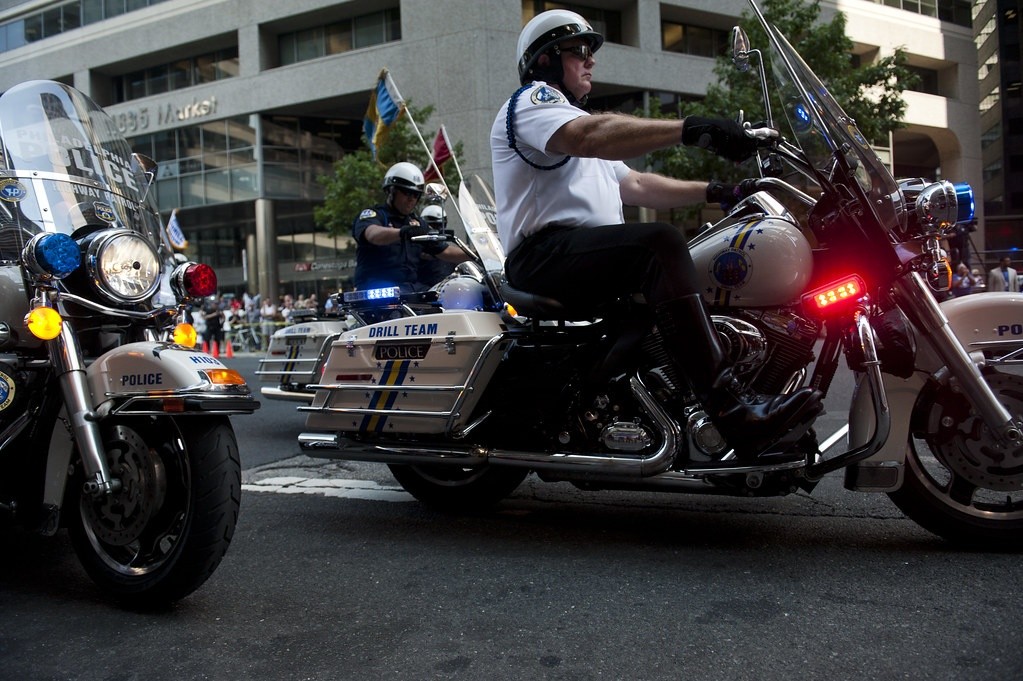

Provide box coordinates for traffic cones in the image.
[200,340,233,359]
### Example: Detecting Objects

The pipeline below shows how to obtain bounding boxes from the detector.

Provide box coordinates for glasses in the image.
[559,45,593,61]
[394,185,423,199]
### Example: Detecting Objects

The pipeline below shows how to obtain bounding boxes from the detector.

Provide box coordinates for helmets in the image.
[419,204,448,233]
[514,8,606,85]
[382,161,426,201]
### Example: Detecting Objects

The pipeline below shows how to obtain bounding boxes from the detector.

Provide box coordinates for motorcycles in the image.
[256,180,510,403]
[291,1,1023,556]
[0,77,262,604]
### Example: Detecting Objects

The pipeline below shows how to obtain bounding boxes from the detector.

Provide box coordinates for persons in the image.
[490,9,823,459]
[986,255,1019,292]
[202,293,317,354]
[351,162,476,321]
[952,264,976,297]
[325,288,342,314]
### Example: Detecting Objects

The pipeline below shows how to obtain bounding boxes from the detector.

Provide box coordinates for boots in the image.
[651,293,823,461]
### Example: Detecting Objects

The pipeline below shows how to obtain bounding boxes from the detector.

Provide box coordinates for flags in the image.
[362,68,406,161]
[166,213,189,249]
[424,129,453,183]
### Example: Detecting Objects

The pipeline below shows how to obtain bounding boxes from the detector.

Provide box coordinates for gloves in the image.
[680,109,782,165]
[705,177,755,218]
[399,225,427,244]
